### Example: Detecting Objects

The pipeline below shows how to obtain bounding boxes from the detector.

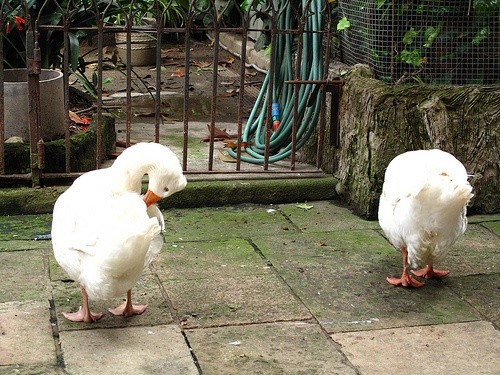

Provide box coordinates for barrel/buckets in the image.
[4,68,65,143]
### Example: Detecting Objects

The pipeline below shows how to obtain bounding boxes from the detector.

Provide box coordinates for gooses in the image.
[377,148,475,288]
[50,141,187,324]
